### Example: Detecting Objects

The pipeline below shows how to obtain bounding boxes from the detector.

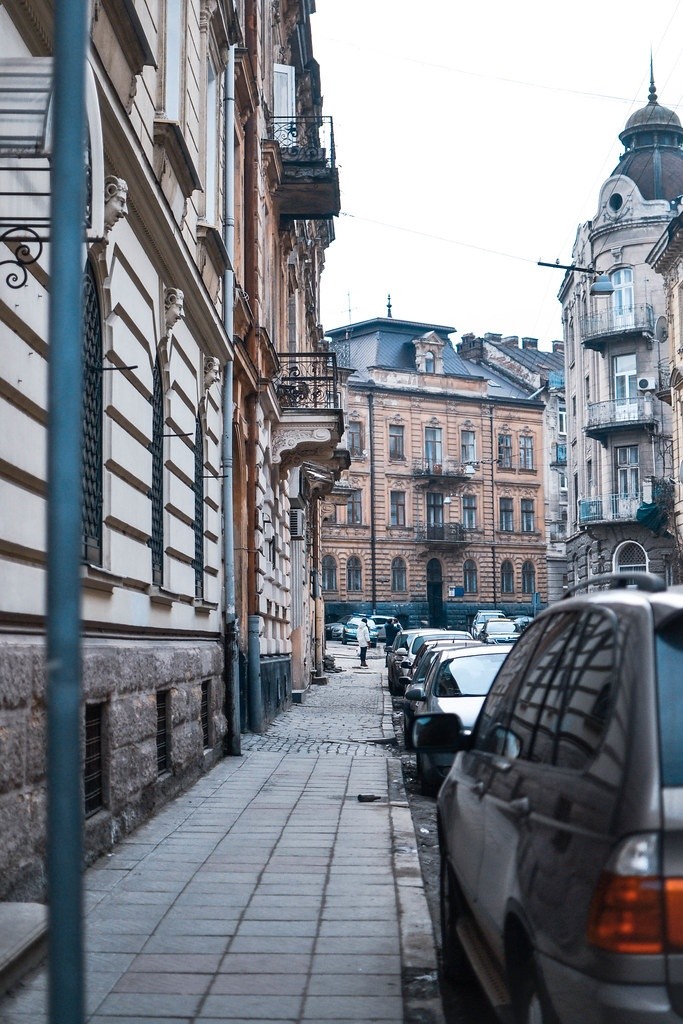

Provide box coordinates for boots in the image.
[361,661,368,668]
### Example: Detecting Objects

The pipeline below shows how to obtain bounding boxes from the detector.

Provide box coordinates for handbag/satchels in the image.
[356,646,361,657]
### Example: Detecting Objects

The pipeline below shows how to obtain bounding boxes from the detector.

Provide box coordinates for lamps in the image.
[464,463,476,474]
[445,496,452,504]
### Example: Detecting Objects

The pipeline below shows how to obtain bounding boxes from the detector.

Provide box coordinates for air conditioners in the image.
[637,377,656,391]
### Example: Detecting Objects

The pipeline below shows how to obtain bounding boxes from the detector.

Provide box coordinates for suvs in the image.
[404,571,682,1024]
[470,610,505,640]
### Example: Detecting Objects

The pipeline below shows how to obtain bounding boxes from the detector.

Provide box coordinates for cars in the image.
[325,613,403,649]
[383,615,534,798]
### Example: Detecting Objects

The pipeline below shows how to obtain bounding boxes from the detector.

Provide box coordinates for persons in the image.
[204,356,219,387]
[163,287,185,328]
[105,175,128,227]
[356,618,371,667]
[385,618,401,668]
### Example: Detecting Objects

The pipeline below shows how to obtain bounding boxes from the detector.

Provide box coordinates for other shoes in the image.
[385,663,388,667]
